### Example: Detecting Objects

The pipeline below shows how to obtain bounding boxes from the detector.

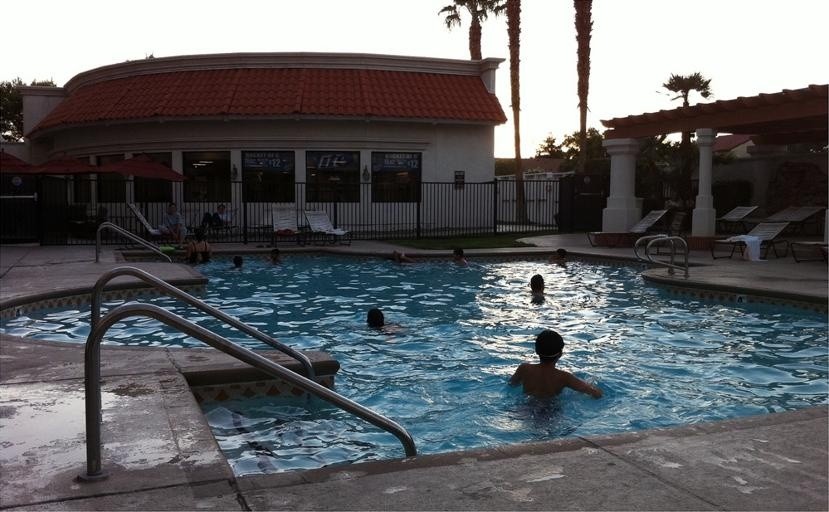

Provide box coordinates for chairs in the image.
[127,204,202,245]
[302,208,352,247]
[588,204,828,263]
[268,204,307,248]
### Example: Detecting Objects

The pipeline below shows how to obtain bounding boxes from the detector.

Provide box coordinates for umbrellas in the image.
[0,150,189,238]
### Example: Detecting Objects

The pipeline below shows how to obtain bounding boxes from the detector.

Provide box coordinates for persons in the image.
[549,248,568,261]
[199,203,232,232]
[162,203,188,249]
[366,308,385,328]
[530,274,545,293]
[453,248,467,265]
[266,248,280,266]
[232,256,243,268]
[509,329,604,400]
[189,229,212,264]
